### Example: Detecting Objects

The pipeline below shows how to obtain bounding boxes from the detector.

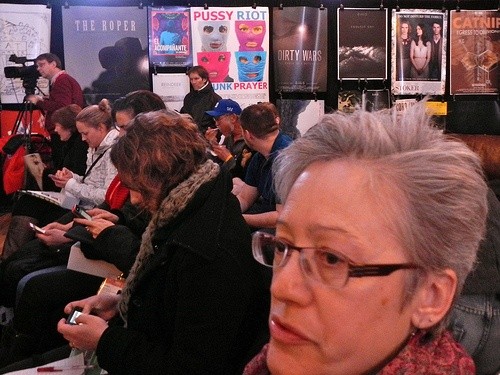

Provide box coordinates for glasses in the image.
[251,231,427,291]
[212,112,235,121]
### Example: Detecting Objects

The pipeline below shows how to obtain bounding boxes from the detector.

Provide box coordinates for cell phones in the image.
[65,306,98,325]
[29,222,45,235]
[48,174,59,180]
[73,204,91,221]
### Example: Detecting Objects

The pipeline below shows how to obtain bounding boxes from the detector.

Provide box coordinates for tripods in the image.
[11,95,45,151]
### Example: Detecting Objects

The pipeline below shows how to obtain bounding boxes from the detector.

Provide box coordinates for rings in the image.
[71,342,76,348]
[85,226,89,230]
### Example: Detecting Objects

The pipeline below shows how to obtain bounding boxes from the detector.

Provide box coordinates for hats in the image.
[205,98,242,117]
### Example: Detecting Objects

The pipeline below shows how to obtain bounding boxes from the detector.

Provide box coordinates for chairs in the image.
[0,266,105,375]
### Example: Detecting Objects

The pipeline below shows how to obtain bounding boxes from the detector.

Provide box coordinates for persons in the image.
[29,53,86,141]
[0,91,487,375]
[179,66,225,142]
[397,21,443,81]
